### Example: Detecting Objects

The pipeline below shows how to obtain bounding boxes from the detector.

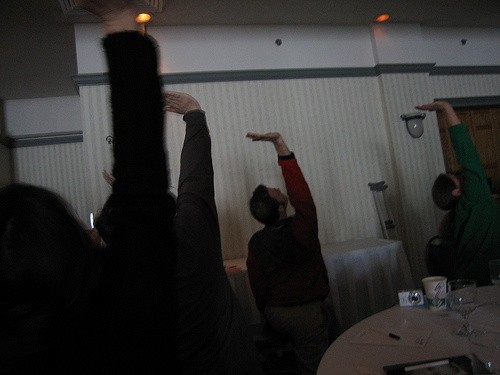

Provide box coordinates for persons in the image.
[245,133,329,375]
[0,0,243,375]
[415,100,500,286]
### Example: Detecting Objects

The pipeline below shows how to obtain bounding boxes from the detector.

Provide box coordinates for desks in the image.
[318,287,500,375]
[225,240,414,330]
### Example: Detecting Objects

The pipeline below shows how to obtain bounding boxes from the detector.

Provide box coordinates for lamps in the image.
[402,113,426,138]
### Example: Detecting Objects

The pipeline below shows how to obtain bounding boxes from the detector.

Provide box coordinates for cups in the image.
[489,259,500,286]
[422,277,447,312]
[467,301,500,375]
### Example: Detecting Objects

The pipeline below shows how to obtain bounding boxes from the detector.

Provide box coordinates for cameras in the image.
[397,287,425,306]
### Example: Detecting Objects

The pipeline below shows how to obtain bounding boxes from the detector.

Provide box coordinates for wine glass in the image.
[448,280,478,336]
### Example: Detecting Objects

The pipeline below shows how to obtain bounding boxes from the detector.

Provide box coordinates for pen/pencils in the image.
[368,326,400,339]
[400,359,450,372]
